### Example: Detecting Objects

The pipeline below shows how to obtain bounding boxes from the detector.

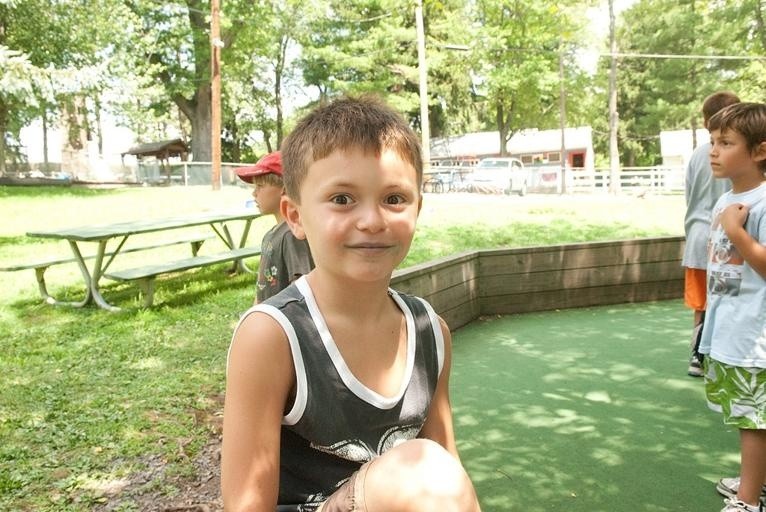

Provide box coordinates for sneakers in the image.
[716,475,766,512]
[688,349,705,377]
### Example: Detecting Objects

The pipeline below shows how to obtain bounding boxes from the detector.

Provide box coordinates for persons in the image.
[230,151,314,308]
[697,103,766,511]
[216,92,485,509]
[682,93,742,378]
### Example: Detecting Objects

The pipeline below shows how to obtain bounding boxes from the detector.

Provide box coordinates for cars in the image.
[471,157,528,197]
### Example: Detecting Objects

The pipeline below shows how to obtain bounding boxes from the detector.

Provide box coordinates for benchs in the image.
[104,249,261,311]
[0,233,216,307]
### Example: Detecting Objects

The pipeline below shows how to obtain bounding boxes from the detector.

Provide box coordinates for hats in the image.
[234,150,285,184]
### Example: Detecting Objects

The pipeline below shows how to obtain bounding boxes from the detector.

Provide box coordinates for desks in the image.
[26,208,262,312]
